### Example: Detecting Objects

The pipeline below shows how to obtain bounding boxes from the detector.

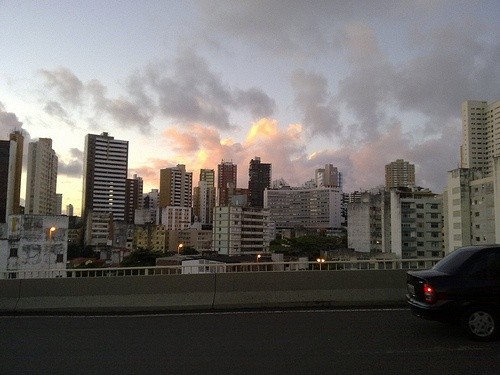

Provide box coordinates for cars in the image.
[406,243,500,342]
